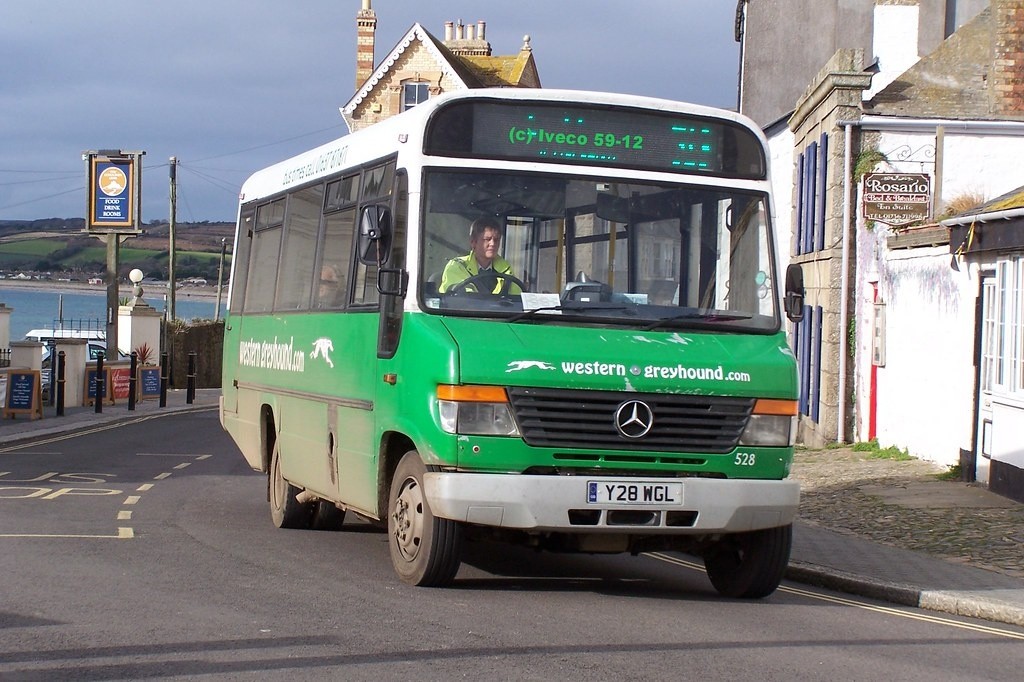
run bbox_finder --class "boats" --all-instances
[20,329,106,354]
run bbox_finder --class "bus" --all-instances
[219,86,805,600]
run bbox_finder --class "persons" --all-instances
[440,218,522,295]
[317,264,348,308]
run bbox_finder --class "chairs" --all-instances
[428,271,443,298]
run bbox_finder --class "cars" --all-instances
[41,341,142,390]
